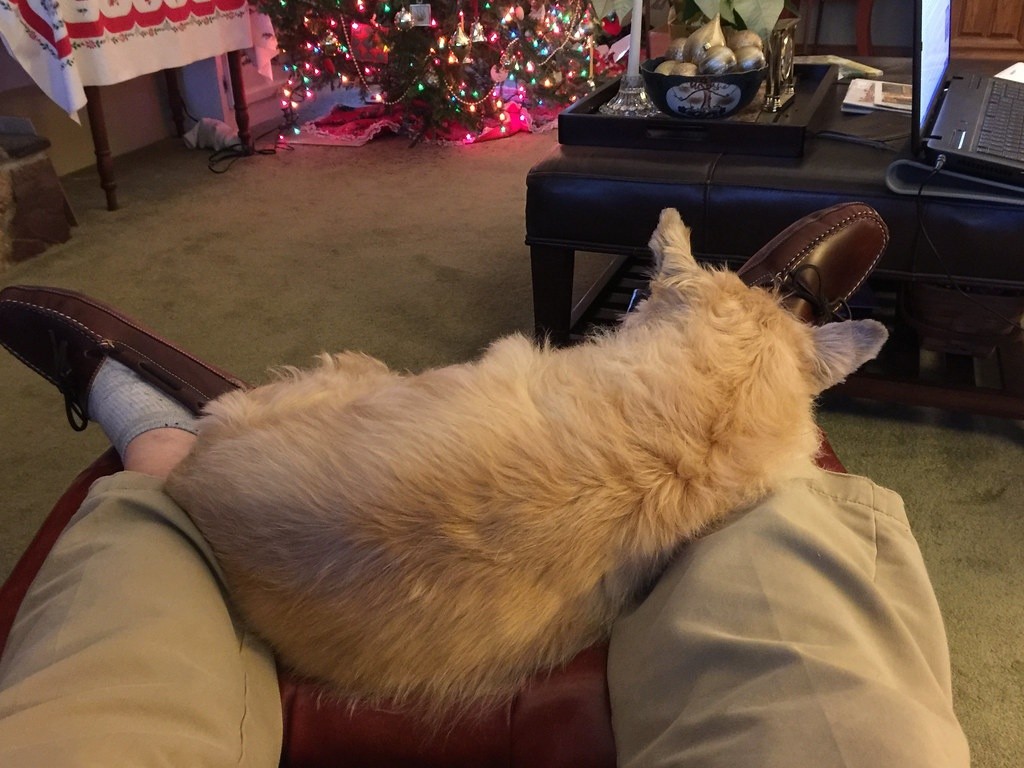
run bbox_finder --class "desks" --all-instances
[0,0,278,212]
[525,55,1024,427]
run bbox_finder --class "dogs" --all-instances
[162,206,890,737]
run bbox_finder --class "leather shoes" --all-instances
[735,199,890,328]
[0,283,256,420]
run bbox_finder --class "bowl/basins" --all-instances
[639,56,769,120]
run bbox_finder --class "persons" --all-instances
[0,201,971,768]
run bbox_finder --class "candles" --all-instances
[626,0,642,75]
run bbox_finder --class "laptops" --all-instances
[911,0,1024,183]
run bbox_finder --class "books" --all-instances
[842,78,913,115]
